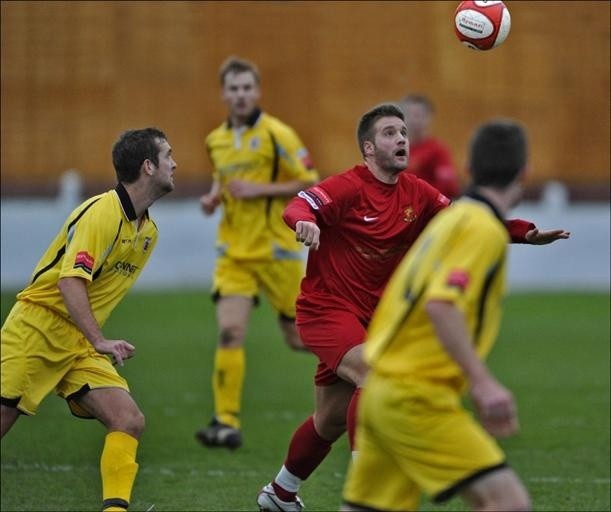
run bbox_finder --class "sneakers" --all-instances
[257,483,306,512]
[195,416,242,449]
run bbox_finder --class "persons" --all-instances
[403,95,460,203]
[195,58,321,447]
[341,118,533,512]
[0,127,178,512]
[256,104,570,512]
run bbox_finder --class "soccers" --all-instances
[452,0,512,51]
